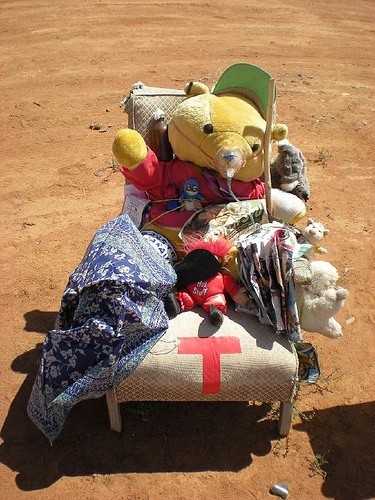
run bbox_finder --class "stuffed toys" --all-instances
[178,178,205,211]
[112,64,306,257]
[294,261,349,338]
[270,143,310,207]
[165,233,256,328]
[298,219,330,256]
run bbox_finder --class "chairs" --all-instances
[104,79,300,438]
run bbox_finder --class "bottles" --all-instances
[151,118,173,161]
